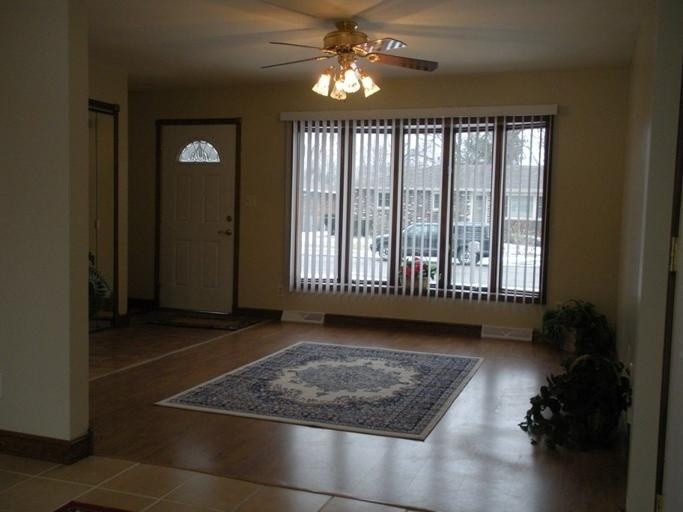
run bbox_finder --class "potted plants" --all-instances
[532,298,617,353]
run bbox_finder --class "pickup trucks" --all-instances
[369,222,490,265]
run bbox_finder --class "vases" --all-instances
[400,274,428,290]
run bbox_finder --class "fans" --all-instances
[261,21,439,72]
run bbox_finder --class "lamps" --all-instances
[311,60,381,101]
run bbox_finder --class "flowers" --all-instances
[397,255,437,281]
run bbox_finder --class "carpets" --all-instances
[143,315,257,331]
[153,339,484,441]
